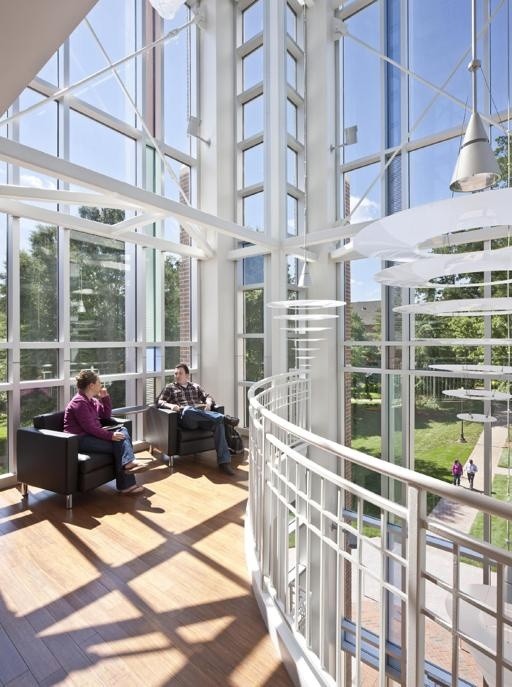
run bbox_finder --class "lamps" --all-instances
[448,1,501,192]
[149,0,187,20]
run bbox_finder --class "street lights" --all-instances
[459,385,467,442]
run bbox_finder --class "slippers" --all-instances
[116,484,146,497]
[120,460,144,472]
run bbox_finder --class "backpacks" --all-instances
[223,425,244,455]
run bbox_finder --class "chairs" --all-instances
[16,408,132,510]
[143,400,224,468]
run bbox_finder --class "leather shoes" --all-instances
[222,415,240,426]
[218,463,236,475]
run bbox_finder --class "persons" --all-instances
[156,362,240,475]
[452,458,462,485]
[465,459,477,489]
[62,369,146,496]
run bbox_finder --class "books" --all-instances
[100,423,125,431]
